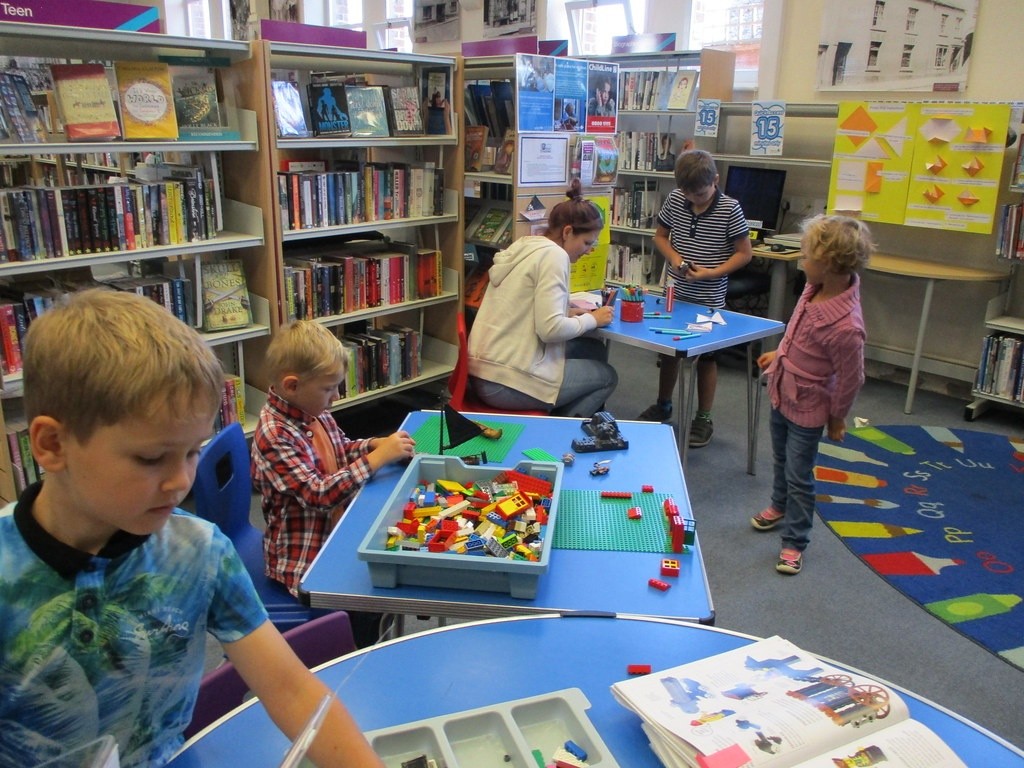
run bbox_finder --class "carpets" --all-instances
[813,425,1024,672]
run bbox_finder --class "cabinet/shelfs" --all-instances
[0,19,1024,502]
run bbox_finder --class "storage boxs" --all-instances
[357,454,564,599]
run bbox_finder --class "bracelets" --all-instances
[678,261,689,273]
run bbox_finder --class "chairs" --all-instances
[192,423,378,658]
[447,312,549,416]
[183,611,358,740]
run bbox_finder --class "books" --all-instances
[604,65,701,294]
[269,65,454,138]
[333,319,427,402]
[0,151,224,264]
[995,135,1024,263]
[283,238,441,322]
[202,367,249,437]
[0,53,242,144]
[277,154,449,231]
[4,409,50,502]
[976,334,1024,402]
[1,256,255,332]
[463,76,517,313]
[610,634,967,768]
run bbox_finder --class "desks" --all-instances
[297,410,715,636]
[566,288,786,477]
[862,253,1012,415]
[167,613,1024,768]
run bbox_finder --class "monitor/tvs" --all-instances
[725,166,787,238]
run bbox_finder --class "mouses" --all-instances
[770,243,786,252]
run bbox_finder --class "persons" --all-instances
[675,76,689,97]
[587,72,618,117]
[1,286,388,768]
[558,103,580,131]
[250,321,417,647]
[495,140,515,173]
[464,178,619,416]
[638,149,753,446]
[656,136,675,172]
[751,212,874,575]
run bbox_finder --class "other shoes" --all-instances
[751,507,785,530]
[776,548,803,574]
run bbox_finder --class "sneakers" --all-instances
[688,417,715,446]
[633,405,673,424]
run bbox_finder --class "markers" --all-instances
[601,279,619,306]
[619,283,648,301]
[643,315,672,318]
[649,327,685,331]
[674,334,701,340]
[596,302,601,308]
[655,331,692,334]
[643,312,660,314]
